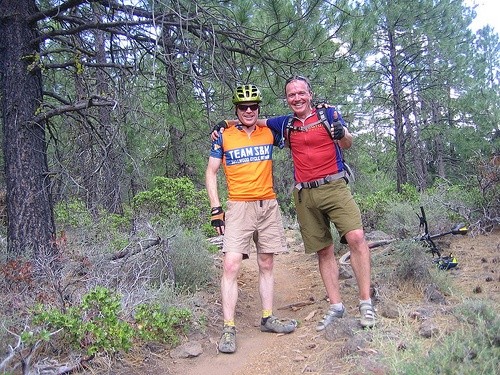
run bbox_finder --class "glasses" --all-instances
[237,104,259,111]
[285,76,311,88]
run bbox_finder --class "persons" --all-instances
[204,83,337,352]
[210,75,376,332]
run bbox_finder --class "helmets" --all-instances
[232,84,263,104]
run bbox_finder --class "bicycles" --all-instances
[338,205,470,271]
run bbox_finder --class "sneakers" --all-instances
[219,326,236,352]
[261,315,294,332]
[359,301,375,326]
[316,304,347,331]
